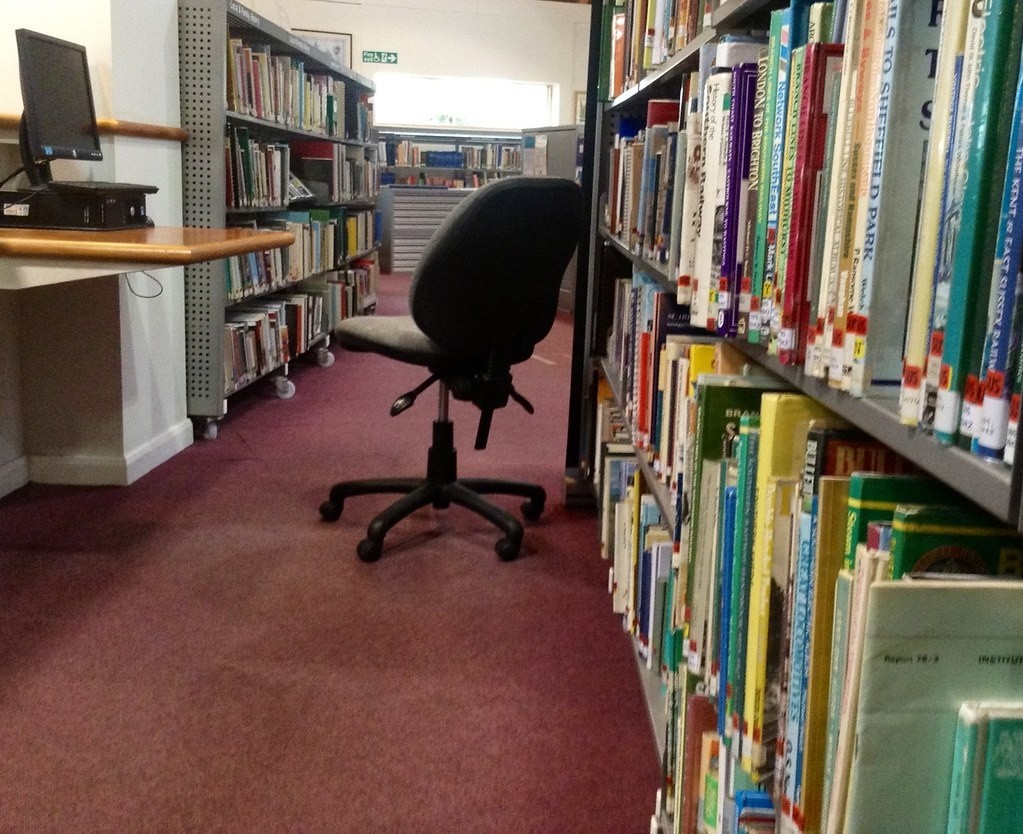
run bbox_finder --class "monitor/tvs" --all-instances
[15,28,104,190]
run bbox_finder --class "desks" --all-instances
[0,223,296,499]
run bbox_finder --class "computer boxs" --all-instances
[0,189,150,232]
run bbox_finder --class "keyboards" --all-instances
[48,180,159,193]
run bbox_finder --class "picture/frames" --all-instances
[291,27,353,68]
[574,90,585,124]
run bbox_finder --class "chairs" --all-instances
[318,173,586,561]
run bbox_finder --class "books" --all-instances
[576,0,1023,834]
[224,22,527,392]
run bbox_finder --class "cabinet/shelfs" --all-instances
[178,0,378,440]
[586,0,1023,834]
[375,124,522,185]
